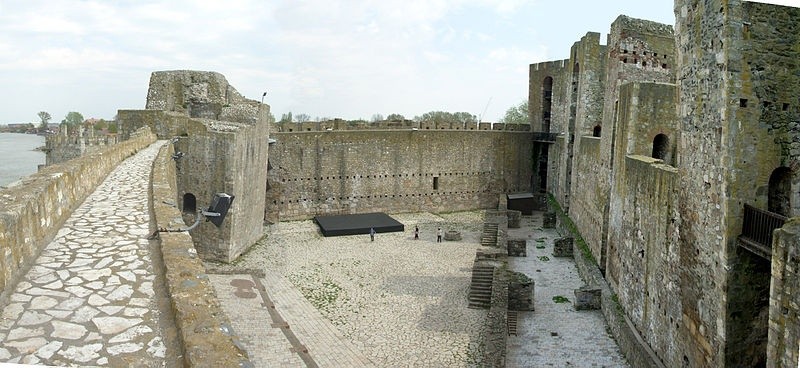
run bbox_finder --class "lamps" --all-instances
[170,137,178,144]
[147,192,236,240]
[170,152,185,161]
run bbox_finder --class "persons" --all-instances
[370,228,376,241]
[437,228,442,243]
[414,225,420,240]
